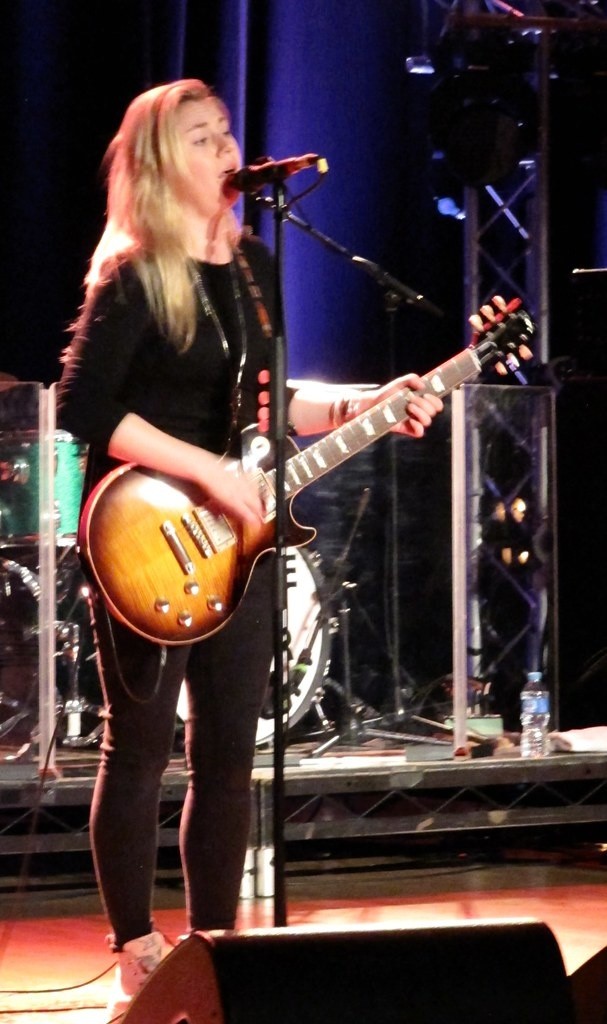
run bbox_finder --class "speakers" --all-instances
[117,921,607,1024]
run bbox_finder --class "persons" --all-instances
[53,81,483,996]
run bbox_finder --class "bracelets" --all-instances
[331,388,359,428]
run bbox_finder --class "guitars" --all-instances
[80,294,536,647]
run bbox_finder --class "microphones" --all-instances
[229,153,325,194]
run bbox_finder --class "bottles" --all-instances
[521,671,551,757]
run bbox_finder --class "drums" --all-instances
[1,427,84,548]
[172,545,334,748]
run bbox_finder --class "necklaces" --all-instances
[185,255,249,450]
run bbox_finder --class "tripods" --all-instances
[286,218,501,764]
[0,552,107,768]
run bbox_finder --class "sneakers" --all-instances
[117,933,176,996]
